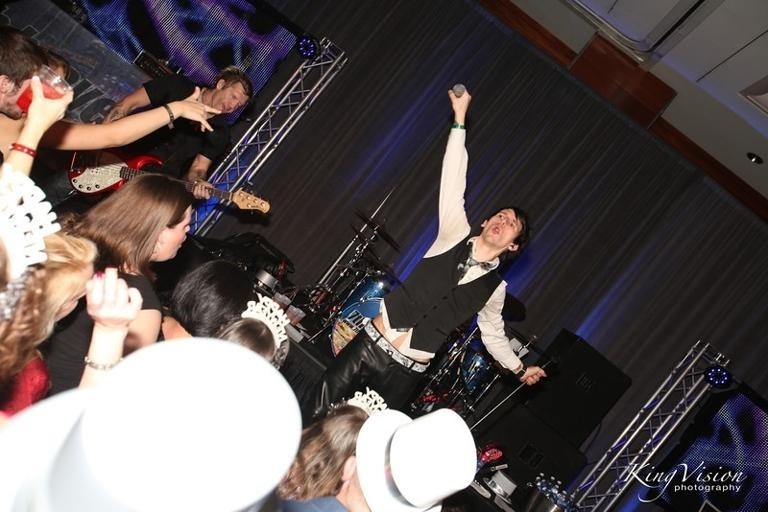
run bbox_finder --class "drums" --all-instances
[332,280,392,356]
[458,352,494,395]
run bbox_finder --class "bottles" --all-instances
[534,472,574,510]
[473,458,486,475]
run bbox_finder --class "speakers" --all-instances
[479,401,588,498]
[525,328,632,444]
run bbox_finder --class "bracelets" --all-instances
[516,366,527,379]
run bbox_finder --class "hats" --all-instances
[355,407,478,512]
[1,337,303,512]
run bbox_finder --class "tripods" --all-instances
[284,227,472,412]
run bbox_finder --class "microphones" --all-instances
[453,83,465,98]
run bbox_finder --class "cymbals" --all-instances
[350,209,399,261]
[510,328,550,360]
[376,263,401,285]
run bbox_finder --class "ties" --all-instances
[458,257,479,281]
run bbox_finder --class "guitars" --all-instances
[68,149,270,214]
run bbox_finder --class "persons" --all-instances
[299,86,548,433]
[0,30,478,512]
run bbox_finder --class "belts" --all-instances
[364,319,431,373]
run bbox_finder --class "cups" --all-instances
[272,292,307,326]
[17,62,75,114]
